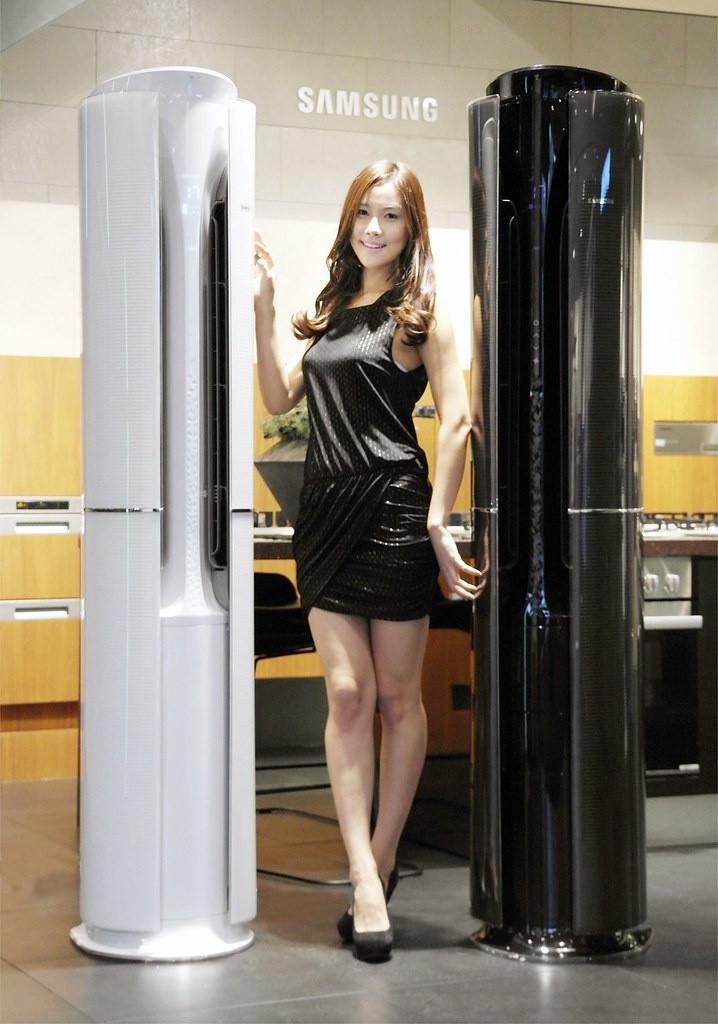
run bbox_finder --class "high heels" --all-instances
[351,875,393,960]
[337,864,400,944]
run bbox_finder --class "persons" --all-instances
[250,158,492,962]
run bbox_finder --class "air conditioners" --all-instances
[71,65,258,963]
[465,66,644,963]
